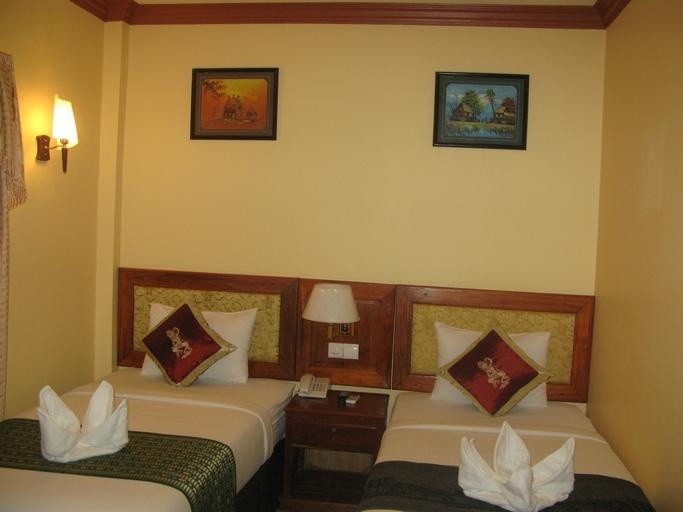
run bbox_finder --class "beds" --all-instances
[362,387,652,512]
[1,366,297,512]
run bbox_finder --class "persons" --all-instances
[223,93,258,123]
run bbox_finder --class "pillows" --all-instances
[143,298,259,385]
[429,317,552,408]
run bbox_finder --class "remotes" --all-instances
[346,394,360,404]
[338,391,350,399]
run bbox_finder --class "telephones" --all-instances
[298,373,330,398]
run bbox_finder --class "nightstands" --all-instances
[281,387,389,511]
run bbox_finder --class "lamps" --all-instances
[301,281,361,340]
[32,95,77,171]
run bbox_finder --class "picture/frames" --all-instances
[191,67,278,141]
[432,69,528,151]
